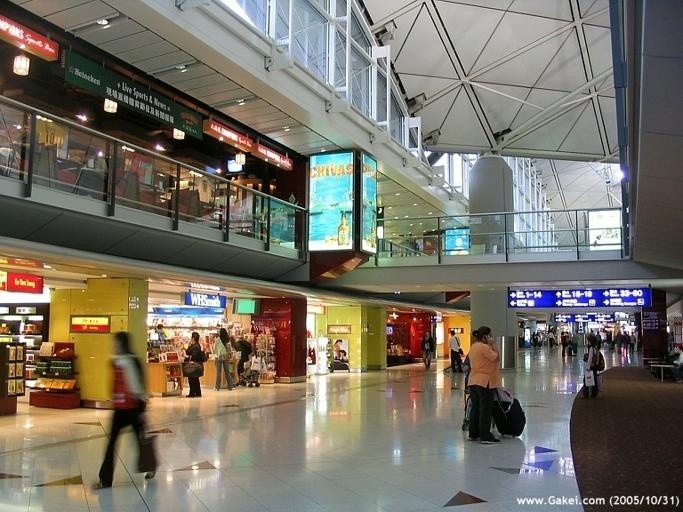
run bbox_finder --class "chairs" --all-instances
[10,143,222,225]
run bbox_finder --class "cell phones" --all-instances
[484,336,488,339]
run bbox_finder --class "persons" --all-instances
[148,326,157,340]
[154,324,165,339]
[397,233,421,256]
[449,331,463,374]
[531,327,635,358]
[229,334,252,387]
[666,332,682,384]
[332,339,342,360]
[200,180,208,202]
[180,332,205,398]
[213,327,236,391]
[420,331,433,369]
[577,333,599,400]
[339,350,347,360]
[466,327,506,446]
[91,332,159,490]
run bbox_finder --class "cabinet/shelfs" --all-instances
[0,303,81,416]
[199,359,240,389]
[144,362,184,397]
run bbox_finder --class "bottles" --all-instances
[370,215,376,248]
[337,209,351,246]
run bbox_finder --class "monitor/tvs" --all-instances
[431,315,442,323]
[232,298,261,315]
[224,158,246,176]
[387,325,394,334]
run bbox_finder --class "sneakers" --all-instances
[480,438,500,444]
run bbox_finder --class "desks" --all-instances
[642,358,676,383]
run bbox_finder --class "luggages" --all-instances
[491,398,525,439]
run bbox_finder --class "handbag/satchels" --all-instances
[584,353,597,363]
[459,349,463,355]
[493,388,513,402]
[182,361,204,377]
[595,351,605,371]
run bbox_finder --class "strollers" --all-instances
[459,372,472,431]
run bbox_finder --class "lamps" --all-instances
[173,127,186,140]
[104,98,118,113]
[235,150,246,165]
[13,51,30,75]
